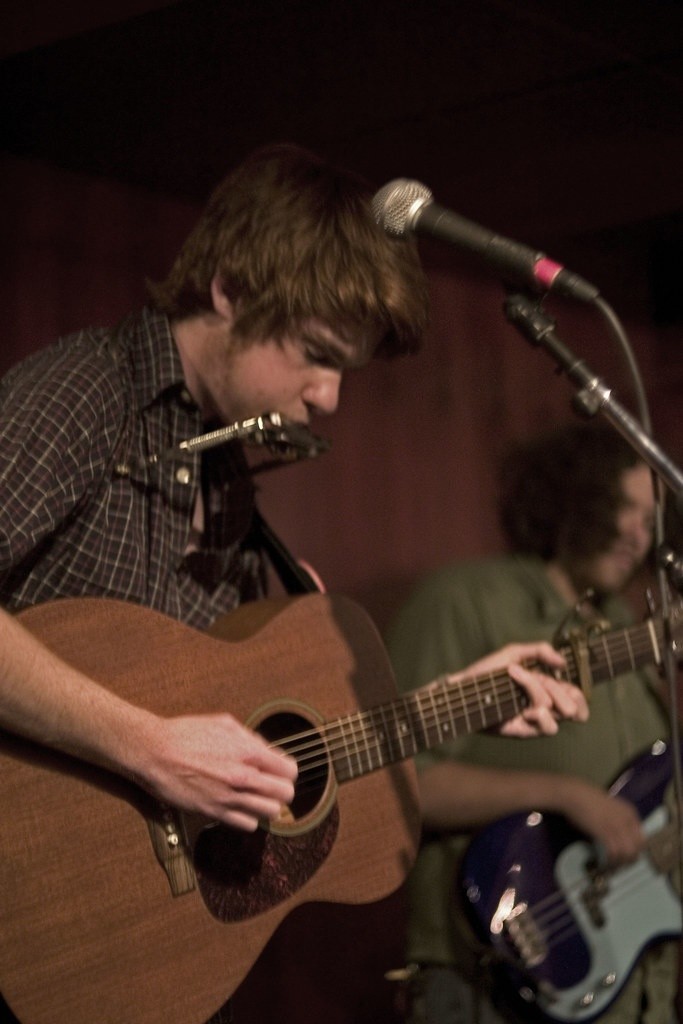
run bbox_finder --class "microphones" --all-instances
[370,179,601,305]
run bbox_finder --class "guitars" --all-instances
[453,736,683,1024]
[1,587,683,1024]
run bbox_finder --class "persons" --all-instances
[0,142,592,830]
[385,424,683,1024]
